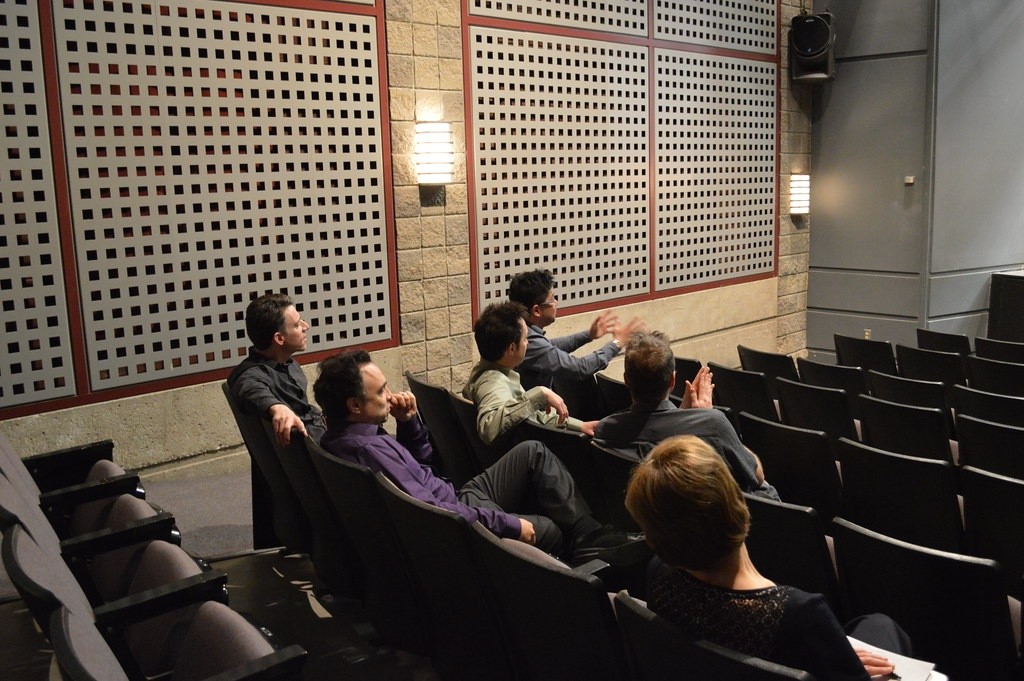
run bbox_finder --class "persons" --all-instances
[229,292,327,447]
[595,330,784,504]
[462,300,601,443]
[624,437,898,680]
[508,270,647,417]
[312,347,657,567]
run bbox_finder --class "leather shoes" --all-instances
[572,526,647,569]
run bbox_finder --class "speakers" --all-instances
[791,13,837,83]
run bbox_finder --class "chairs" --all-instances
[3,249,1024,681]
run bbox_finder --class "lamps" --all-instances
[410,120,457,190]
[787,172,813,218]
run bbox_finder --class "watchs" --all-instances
[610,339,624,350]
